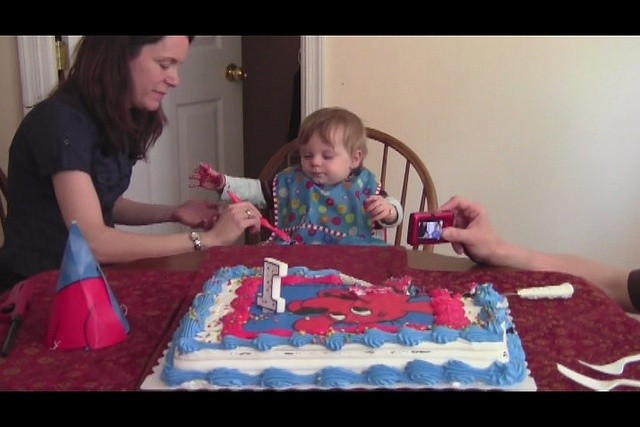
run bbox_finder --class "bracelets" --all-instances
[189,231,202,251]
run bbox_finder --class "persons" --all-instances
[435,195,639,315]
[1,35,261,290]
[189,107,403,247]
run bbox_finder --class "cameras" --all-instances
[406,211,457,246]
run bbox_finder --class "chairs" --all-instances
[0,163,15,248]
[241,128,438,253]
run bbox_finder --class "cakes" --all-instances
[161,264,528,386]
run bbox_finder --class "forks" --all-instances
[576,354,640,377]
[556,362,640,392]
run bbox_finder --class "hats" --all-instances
[45,221,129,353]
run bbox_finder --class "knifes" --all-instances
[500,281,575,300]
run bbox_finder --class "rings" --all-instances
[245,209,251,217]
